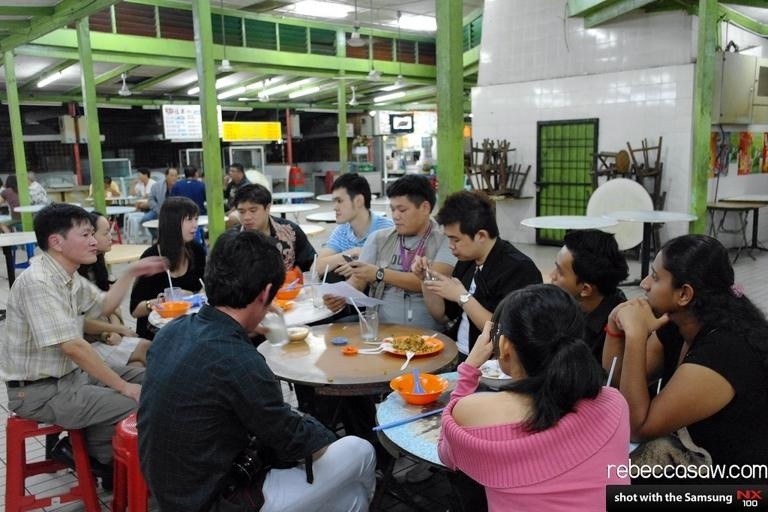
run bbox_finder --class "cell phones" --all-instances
[342,253,357,268]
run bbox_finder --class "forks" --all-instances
[400,350,414,371]
[359,337,395,354]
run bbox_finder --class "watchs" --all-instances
[456,292,472,308]
[375,268,386,283]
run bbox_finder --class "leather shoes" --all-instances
[49,439,98,485]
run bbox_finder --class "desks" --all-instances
[1,180,387,309]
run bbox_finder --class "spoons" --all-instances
[413,368,425,393]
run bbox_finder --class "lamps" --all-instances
[366,0,384,81]
[346,0,369,47]
[215,1,234,73]
[1,53,364,109]
[390,7,410,88]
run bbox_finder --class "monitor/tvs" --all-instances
[391,114,414,133]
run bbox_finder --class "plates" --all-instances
[384,336,445,357]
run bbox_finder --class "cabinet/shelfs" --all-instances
[584,178,659,252]
[711,47,722,125]
[751,58,768,125]
[726,193,768,252]
[520,209,618,244]
[721,52,759,126]
[602,206,701,291]
[710,201,761,267]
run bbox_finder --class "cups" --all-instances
[157,287,182,304]
[311,283,327,309]
[262,306,291,348]
[303,271,318,299]
[359,310,379,341]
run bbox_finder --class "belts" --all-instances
[5,377,56,389]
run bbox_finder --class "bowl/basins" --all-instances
[157,302,190,318]
[276,283,302,300]
[286,324,311,343]
[284,270,298,283]
[480,360,513,390]
[388,373,449,404]
[421,273,450,283]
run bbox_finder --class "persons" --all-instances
[1,164,255,255]
[602,233,768,509]
[323,174,458,330]
[548,226,628,371]
[129,194,206,342]
[435,281,633,511]
[73,212,155,367]
[1,203,169,496]
[314,174,395,279]
[408,187,543,374]
[135,228,376,510]
[228,184,317,272]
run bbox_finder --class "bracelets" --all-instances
[602,323,625,340]
[145,300,153,314]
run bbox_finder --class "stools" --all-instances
[460,128,531,199]
[108,403,158,511]
[5,412,101,511]
[590,130,673,260]
